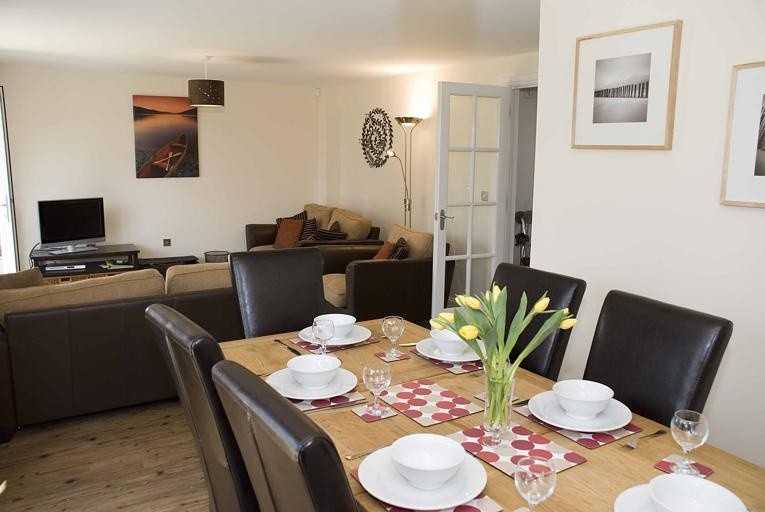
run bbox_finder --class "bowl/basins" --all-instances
[430,328,468,355]
[390,433,466,490]
[314,314,356,340]
[286,354,342,390]
[649,474,726,511]
[552,380,615,420]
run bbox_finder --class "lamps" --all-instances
[386,116,423,228]
[187,79,225,108]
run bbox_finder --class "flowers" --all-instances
[431,288,577,379]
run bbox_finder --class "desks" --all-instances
[31,244,140,277]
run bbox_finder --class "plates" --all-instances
[416,338,487,362]
[357,445,488,510]
[266,368,358,400]
[299,325,372,346]
[614,483,748,511]
[528,390,633,432]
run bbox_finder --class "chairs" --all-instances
[211,359,362,511]
[145,300,261,512]
[230,248,328,338]
[582,288,735,429]
[488,265,586,380]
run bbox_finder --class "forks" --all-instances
[616,429,668,448]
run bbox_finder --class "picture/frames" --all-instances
[720,61,765,207]
[571,20,682,151]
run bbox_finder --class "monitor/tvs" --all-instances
[37,196,106,254]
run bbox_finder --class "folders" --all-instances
[104,257,134,270]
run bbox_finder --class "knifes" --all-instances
[512,399,531,407]
[274,339,301,356]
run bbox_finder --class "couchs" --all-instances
[314,244,456,322]
[5,262,246,432]
[0,325,19,444]
[245,208,384,251]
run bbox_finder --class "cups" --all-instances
[515,456,557,511]
[381,316,404,357]
[312,320,335,356]
[362,362,391,417]
[670,409,708,474]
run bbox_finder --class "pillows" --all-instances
[273,220,304,248]
[276,210,306,230]
[389,238,409,258]
[0,268,46,289]
[310,229,347,241]
[300,218,316,238]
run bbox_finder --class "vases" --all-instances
[483,379,516,432]
